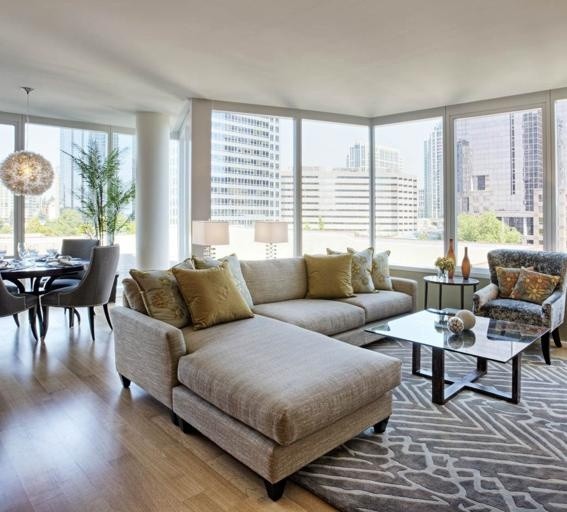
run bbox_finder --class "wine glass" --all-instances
[16,241,39,259]
[0,244,8,260]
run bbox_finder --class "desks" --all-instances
[423,275,480,316]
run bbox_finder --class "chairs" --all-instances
[474,249,567,372]
[0,237,123,347]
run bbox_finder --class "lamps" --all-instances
[190,220,231,259]
[1,85,55,199]
[252,221,289,255]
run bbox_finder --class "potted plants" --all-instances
[53,130,136,303]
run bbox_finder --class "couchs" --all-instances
[108,256,420,500]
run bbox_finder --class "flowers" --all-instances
[435,256,455,272]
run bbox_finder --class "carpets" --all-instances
[257,317,566,511]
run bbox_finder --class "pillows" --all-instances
[303,253,356,299]
[325,247,381,294]
[509,264,560,306]
[494,264,534,300]
[121,249,257,331]
[346,246,398,292]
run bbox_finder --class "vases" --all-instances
[439,269,446,278]
